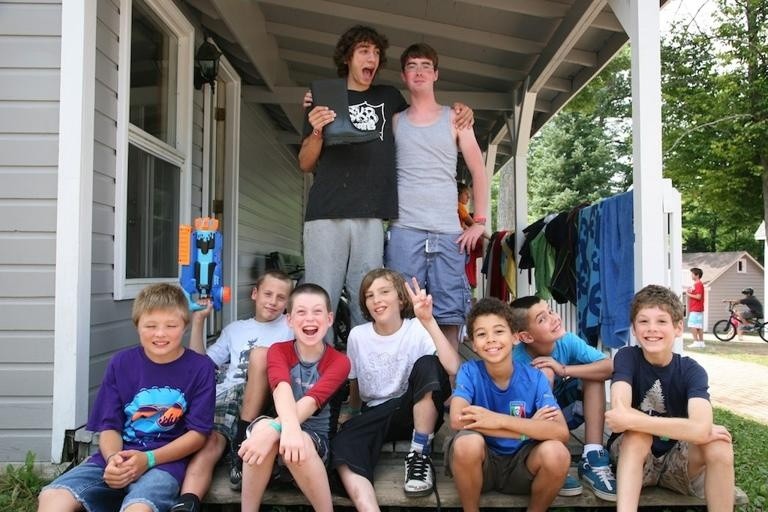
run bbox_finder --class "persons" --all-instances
[331,269,459,511]
[382,44,488,354]
[175,272,293,512]
[441,298,570,511]
[36,285,215,512]
[453,182,480,288]
[605,287,734,512]
[510,296,620,502]
[297,25,408,355]
[685,270,708,349]
[237,284,350,512]
[721,288,763,334]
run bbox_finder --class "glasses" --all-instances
[401,62,437,73]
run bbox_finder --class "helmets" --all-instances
[742,287,755,294]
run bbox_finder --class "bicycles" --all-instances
[713,301,768,342]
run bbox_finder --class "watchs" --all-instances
[472,214,487,224]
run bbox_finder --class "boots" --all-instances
[309,78,380,148]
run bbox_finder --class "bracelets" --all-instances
[519,433,528,442]
[660,435,669,441]
[266,420,281,434]
[145,450,157,468]
[561,364,571,383]
[346,406,362,415]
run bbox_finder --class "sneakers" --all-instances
[228,456,246,491]
[578,447,620,502]
[403,449,437,497]
[741,327,754,334]
[686,342,707,349]
[754,323,763,331]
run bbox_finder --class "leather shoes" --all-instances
[558,475,584,498]
